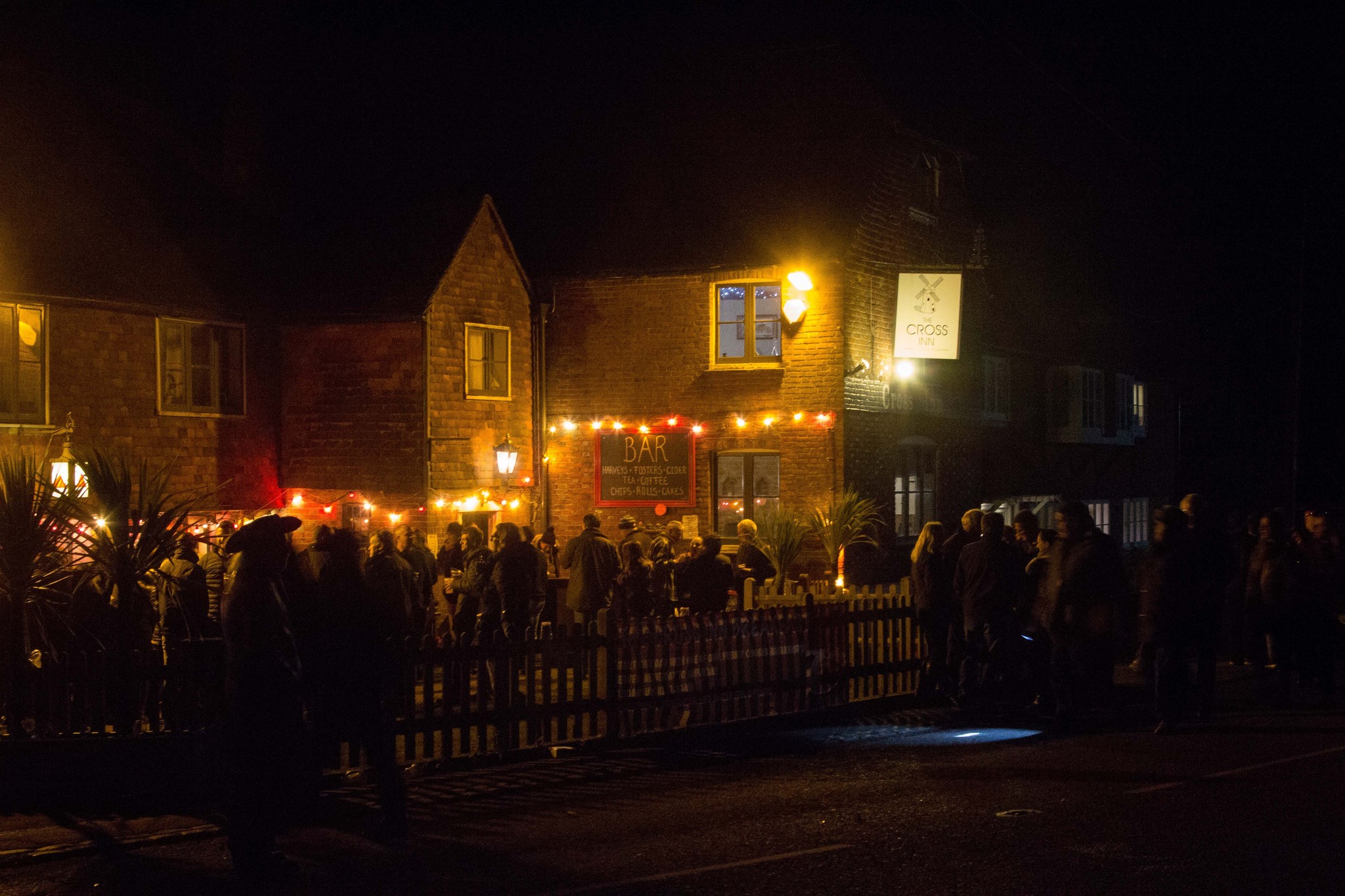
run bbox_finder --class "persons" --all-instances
[226,515,309,884]
[844,482,1345,743]
[311,531,409,840]
[18,501,780,733]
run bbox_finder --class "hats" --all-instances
[541,526,556,545]
[617,515,636,529]
[225,514,301,555]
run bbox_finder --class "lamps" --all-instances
[492,433,521,488]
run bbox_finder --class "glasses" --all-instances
[490,534,500,540]
